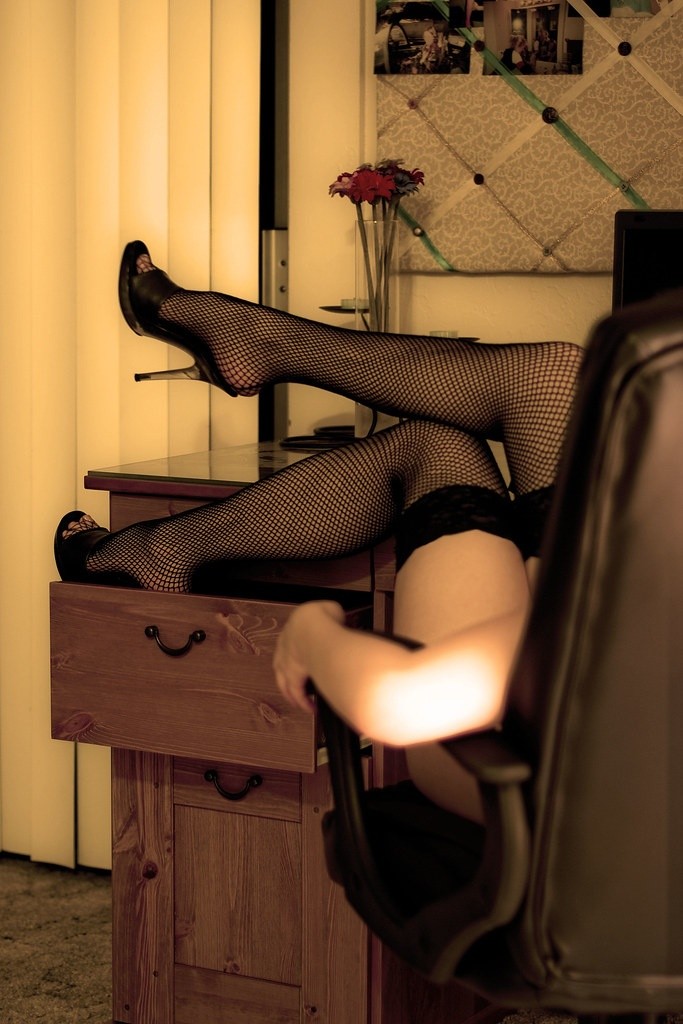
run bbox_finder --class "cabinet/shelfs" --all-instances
[50,438,479,1024]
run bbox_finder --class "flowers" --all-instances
[328,158,426,332]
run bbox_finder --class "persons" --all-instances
[401,27,440,74]
[53,241,585,828]
[488,36,537,75]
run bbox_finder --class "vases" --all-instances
[353,220,398,438]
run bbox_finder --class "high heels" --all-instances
[52,510,143,590]
[119,240,248,397]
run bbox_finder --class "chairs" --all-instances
[307,290,683,1024]
